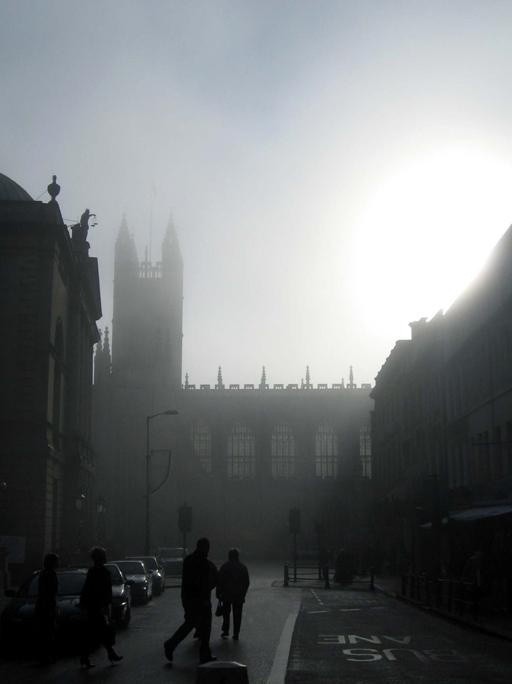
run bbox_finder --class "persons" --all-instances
[216,549,249,640]
[163,538,217,663]
[39,554,58,665]
[80,549,123,668]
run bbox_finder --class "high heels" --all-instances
[107,648,123,665]
[79,656,96,669]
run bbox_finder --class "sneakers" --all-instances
[221,631,240,640]
[199,656,217,663]
[163,641,174,662]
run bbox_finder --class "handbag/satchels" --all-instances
[215,600,224,617]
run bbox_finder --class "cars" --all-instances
[0,548,188,650]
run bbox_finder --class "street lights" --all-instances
[145,410,178,554]
